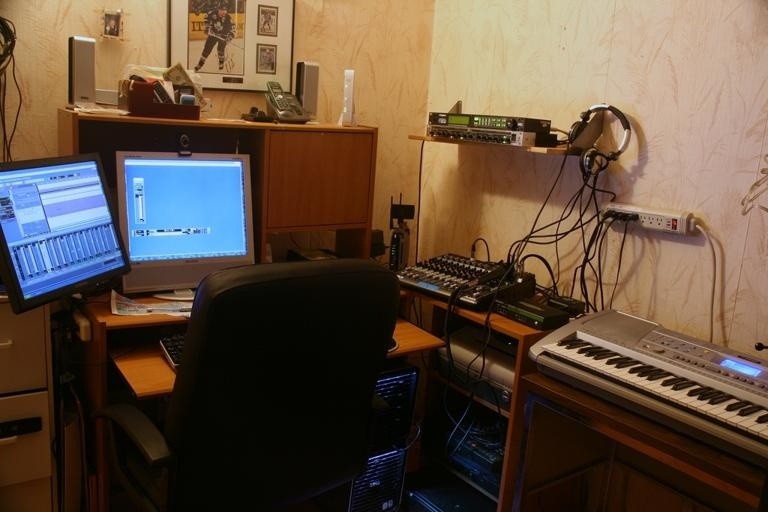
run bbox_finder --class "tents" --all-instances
[568,103,631,175]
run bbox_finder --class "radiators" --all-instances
[153,80,174,104]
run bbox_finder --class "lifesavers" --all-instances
[347,363,419,512]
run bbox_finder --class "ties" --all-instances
[0,153,131,314]
[116,151,256,301]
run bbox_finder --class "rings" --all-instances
[146,307,192,312]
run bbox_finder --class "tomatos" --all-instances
[528,310,768,470]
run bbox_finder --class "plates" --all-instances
[396,278,548,512]
[0,296,57,512]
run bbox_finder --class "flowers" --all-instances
[455,261,513,292]
[755,343,768,351]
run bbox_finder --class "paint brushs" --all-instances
[601,203,692,235]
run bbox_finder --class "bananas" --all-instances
[160,331,188,370]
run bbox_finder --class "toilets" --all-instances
[265,82,311,124]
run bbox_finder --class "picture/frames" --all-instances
[58,106,445,512]
[518,374,768,512]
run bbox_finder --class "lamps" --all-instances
[97,258,401,512]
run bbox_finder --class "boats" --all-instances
[295,61,320,124]
[68,36,95,110]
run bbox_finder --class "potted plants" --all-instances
[97,8,126,43]
[167,0,296,93]
